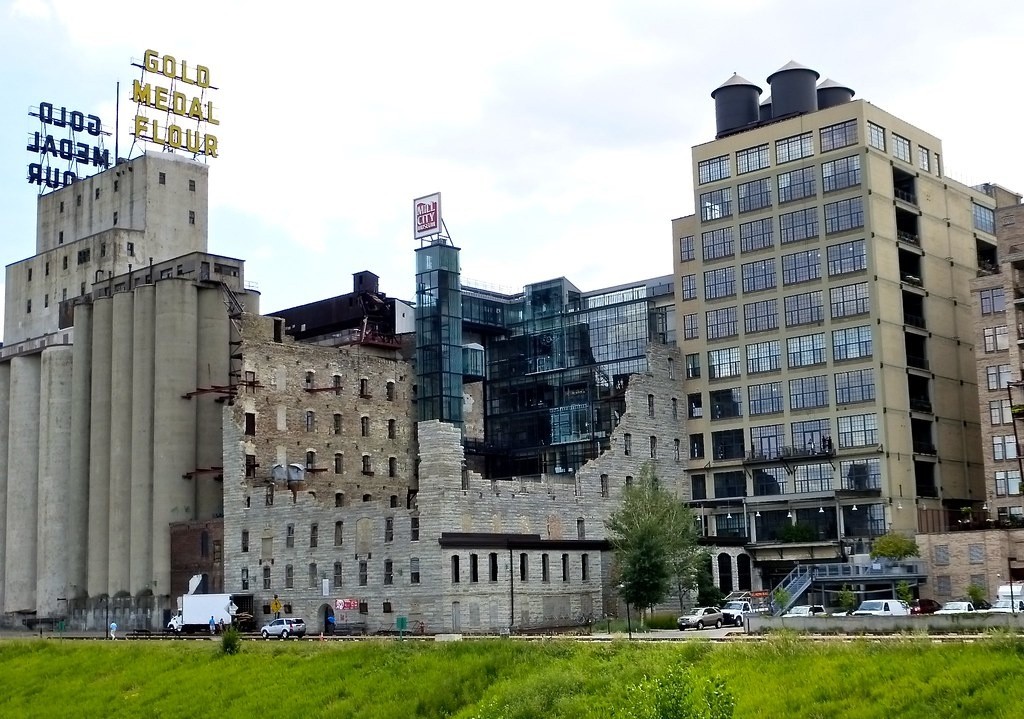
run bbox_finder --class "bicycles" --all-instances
[577,612,597,625]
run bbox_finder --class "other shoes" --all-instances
[114,637,115,640]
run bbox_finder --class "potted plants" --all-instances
[869,532,921,574]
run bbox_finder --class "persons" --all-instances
[208,615,216,636]
[328,613,337,636]
[218,618,225,636]
[109,621,117,641]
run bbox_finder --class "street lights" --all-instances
[99,594,109,638]
[619,581,634,640]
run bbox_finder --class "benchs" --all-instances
[133,629,151,640]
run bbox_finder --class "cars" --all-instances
[987,600,1024,612]
[934,602,978,614]
[910,599,943,614]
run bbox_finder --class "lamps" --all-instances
[897,502,903,510]
[818,507,824,513]
[696,515,701,521]
[851,504,858,510]
[756,511,761,516]
[727,513,732,518]
[983,500,989,510]
[922,503,927,511]
[786,512,793,517]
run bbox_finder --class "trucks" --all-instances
[167,593,253,634]
[997,584,1024,600]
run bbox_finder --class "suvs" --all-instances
[677,607,723,630]
[261,618,306,638]
[721,601,752,626]
[852,600,911,615]
[782,605,827,617]
